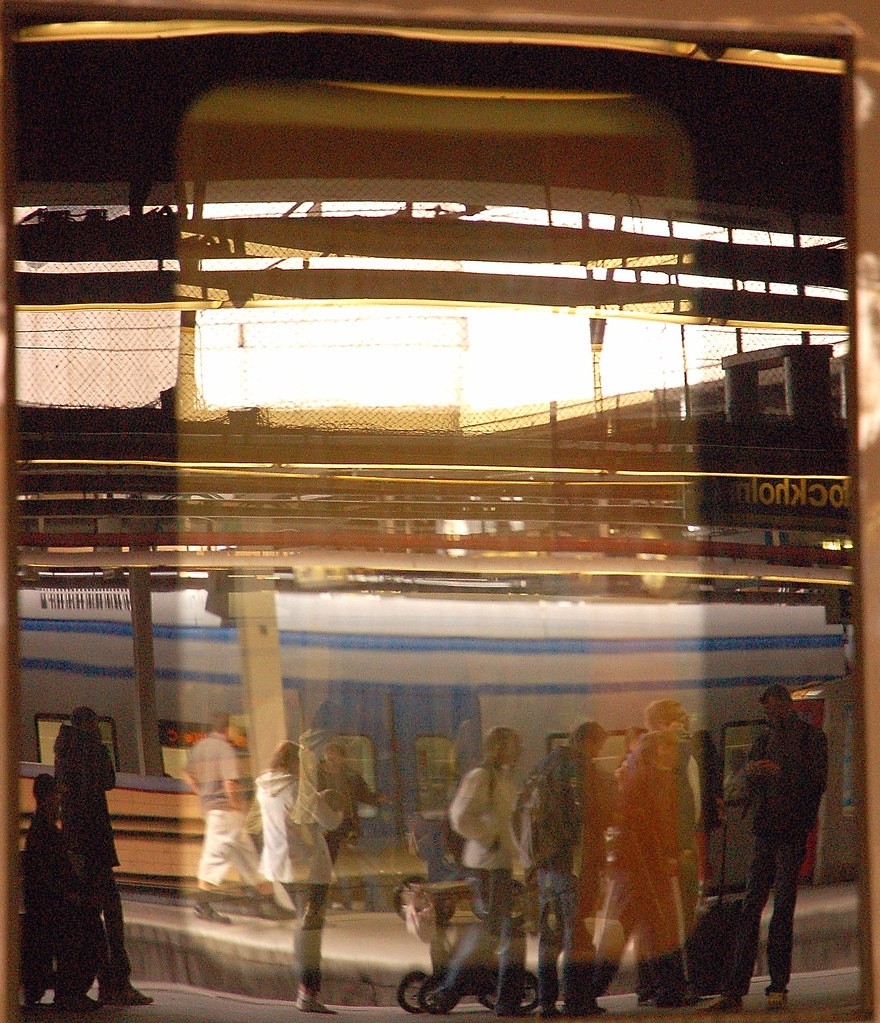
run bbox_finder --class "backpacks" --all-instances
[510,754,578,866]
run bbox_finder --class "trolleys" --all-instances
[396,880,540,1016]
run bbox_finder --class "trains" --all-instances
[9,564,860,844]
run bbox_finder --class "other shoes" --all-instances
[297,995,336,1014]
[767,990,787,1008]
[51,989,98,1011]
[494,1005,536,1016]
[194,904,231,923]
[702,998,741,1012]
[98,985,152,1005]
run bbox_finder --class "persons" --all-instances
[179,705,391,1014]
[435,686,828,1018]
[23,707,154,1014]
